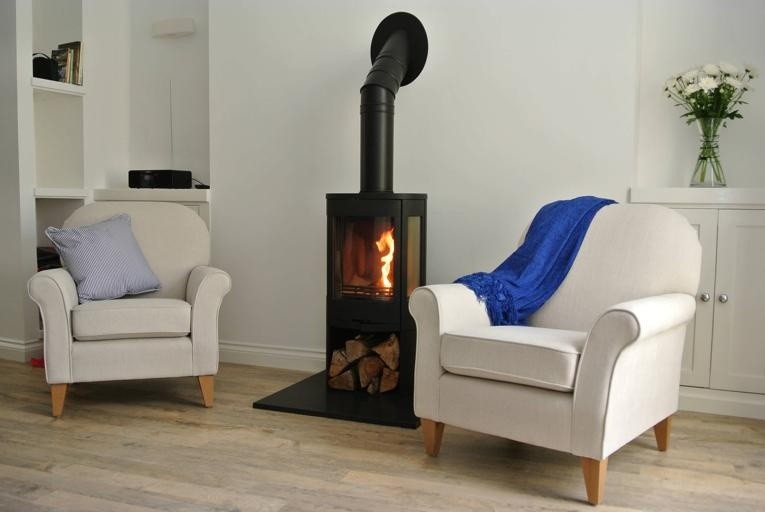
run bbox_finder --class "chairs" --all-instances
[27,201,232,417]
[408,203,703,506]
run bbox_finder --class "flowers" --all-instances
[663,61,757,183]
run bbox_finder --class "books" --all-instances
[50,41,81,84]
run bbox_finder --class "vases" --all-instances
[690,118,726,187]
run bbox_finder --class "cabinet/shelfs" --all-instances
[670,202,765,419]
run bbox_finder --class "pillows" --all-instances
[44,213,161,303]
